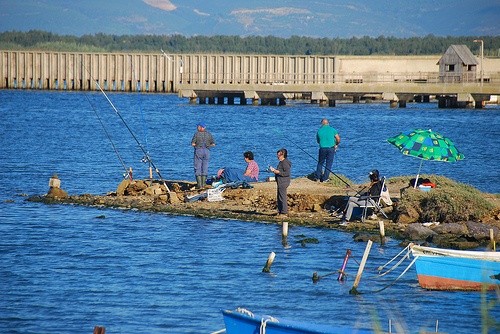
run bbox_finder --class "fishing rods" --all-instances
[71,63,170,194]
[286,137,359,193]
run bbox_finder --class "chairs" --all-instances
[357,176,389,224]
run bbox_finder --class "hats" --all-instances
[196,121,205,128]
[368,169,379,175]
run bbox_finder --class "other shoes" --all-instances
[316,178,330,183]
[274,213,287,218]
[339,221,348,226]
[336,212,344,218]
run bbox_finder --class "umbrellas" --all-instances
[386,129,465,190]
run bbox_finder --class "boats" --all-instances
[219,308,383,334]
[409,243,500,291]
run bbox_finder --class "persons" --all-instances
[243,151,259,182]
[336,169,381,226]
[314,119,340,182]
[192,123,215,188]
[273,149,292,219]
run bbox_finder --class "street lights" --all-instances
[473,39,484,86]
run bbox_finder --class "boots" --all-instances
[194,176,207,188]
